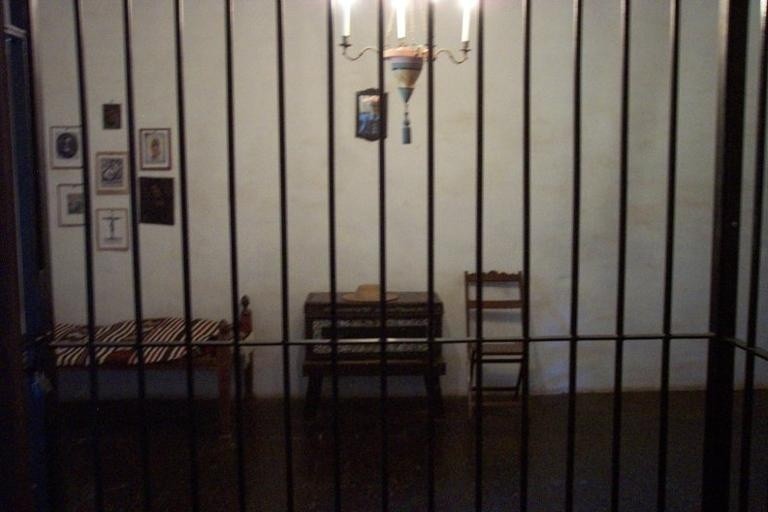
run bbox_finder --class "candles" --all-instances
[331,1,481,43]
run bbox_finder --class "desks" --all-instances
[300,290,447,424]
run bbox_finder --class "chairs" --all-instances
[465,271,534,425]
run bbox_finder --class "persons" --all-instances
[359,94,382,136]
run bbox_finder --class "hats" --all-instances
[342,283,398,302]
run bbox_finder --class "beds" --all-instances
[21,297,256,451]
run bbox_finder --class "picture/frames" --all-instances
[46,101,176,251]
[355,87,388,142]
